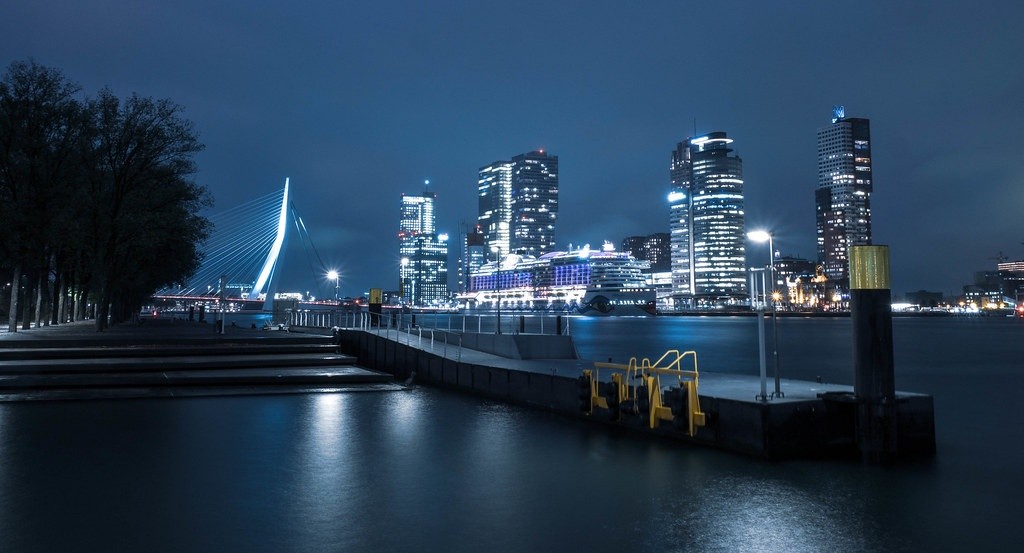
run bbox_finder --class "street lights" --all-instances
[746,226,782,397]
[326,269,339,305]
[400,257,409,295]
[490,245,502,335]
